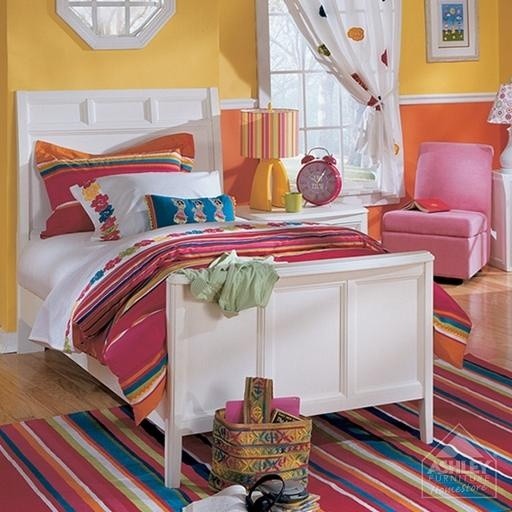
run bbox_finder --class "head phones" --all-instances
[245,473,285,512]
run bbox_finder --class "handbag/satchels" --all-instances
[207,377,312,494]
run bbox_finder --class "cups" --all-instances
[285,192,302,214]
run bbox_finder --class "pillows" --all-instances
[32,131,236,243]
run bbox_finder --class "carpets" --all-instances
[2,352,511,512]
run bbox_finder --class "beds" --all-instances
[13,87,433,489]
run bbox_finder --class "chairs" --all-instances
[381,140,494,284]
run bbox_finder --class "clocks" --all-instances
[296,146,343,208]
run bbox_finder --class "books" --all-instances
[400,198,451,213]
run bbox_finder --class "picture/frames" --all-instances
[423,1,480,64]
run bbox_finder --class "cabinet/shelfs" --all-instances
[489,169,511,272]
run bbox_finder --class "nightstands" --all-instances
[233,203,369,238]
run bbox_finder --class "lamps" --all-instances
[237,103,302,211]
[486,82,512,169]
